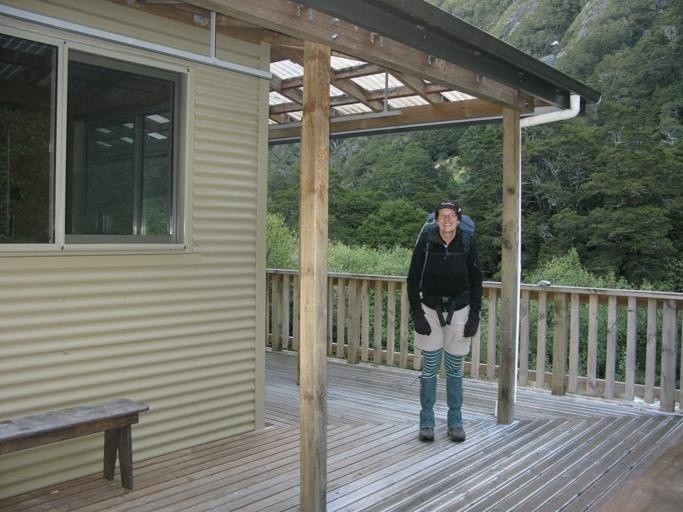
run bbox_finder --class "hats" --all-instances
[435,198,462,220]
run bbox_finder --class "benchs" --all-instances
[1,397,151,491]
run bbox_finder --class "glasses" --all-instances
[438,214,456,218]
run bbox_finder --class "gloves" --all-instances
[464,312,478,338]
[410,311,431,335]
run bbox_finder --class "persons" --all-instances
[407,199,482,441]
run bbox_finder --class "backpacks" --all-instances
[426,212,475,235]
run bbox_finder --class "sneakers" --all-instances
[419,428,434,441]
[449,428,465,440]
